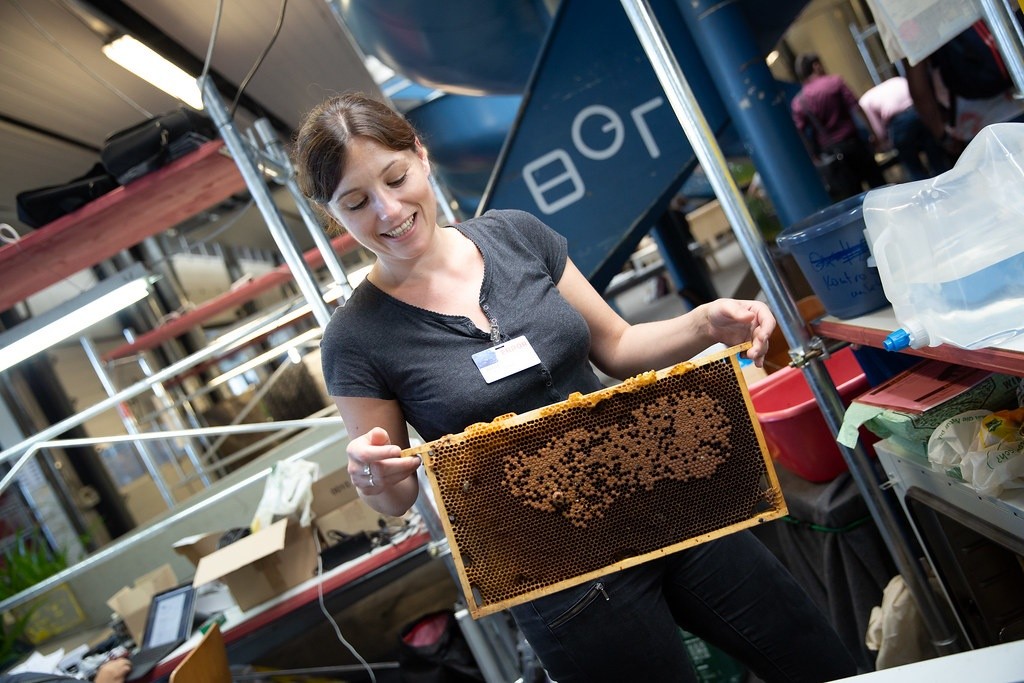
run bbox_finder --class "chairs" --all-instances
[167,622,231,683]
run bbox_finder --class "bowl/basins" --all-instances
[749,345,881,481]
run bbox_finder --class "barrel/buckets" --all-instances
[774,182,896,320]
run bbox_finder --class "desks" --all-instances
[607,242,721,305]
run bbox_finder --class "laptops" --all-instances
[122,580,199,683]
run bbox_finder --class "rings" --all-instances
[369,475,375,487]
[363,464,370,475]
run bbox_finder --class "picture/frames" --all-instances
[9,582,87,647]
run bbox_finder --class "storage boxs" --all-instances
[105,563,179,647]
[751,347,878,482]
[305,461,406,545]
[192,519,318,612]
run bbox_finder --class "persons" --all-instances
[858,77,945,181]
[791,54,886,198]
[294,93,860,683]
[1,657,133,683]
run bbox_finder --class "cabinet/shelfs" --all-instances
[0,75,522,683]
[615,0,1024,656]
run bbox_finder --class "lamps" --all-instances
[100,29,213,110]
[0,261,166,373]
